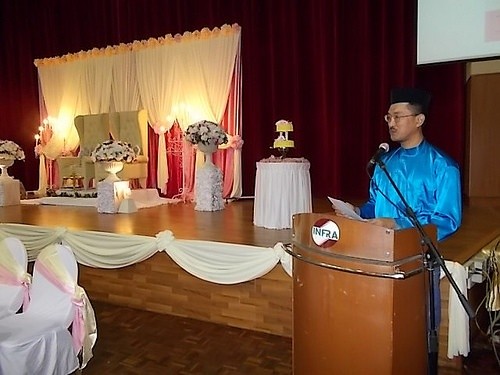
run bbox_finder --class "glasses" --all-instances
[384,113,418,123]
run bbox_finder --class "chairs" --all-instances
[0,237,32,320]
[56,110,148,190]
[0,244,97,375]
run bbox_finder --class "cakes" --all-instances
[275,120,293,131]
[273,136,294,147]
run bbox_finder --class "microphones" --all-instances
[367,143,390,170]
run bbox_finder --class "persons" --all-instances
[332,85,463,375]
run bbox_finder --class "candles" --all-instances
[34,116,56,147]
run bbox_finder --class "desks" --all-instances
[253,161,312,230]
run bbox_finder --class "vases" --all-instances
[100,161,124,174]
[0,159,15,168]
[197,142,218,153]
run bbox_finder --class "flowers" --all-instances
[89,140,137,165]
[0,140,25,161]
[184,120,228,146]
